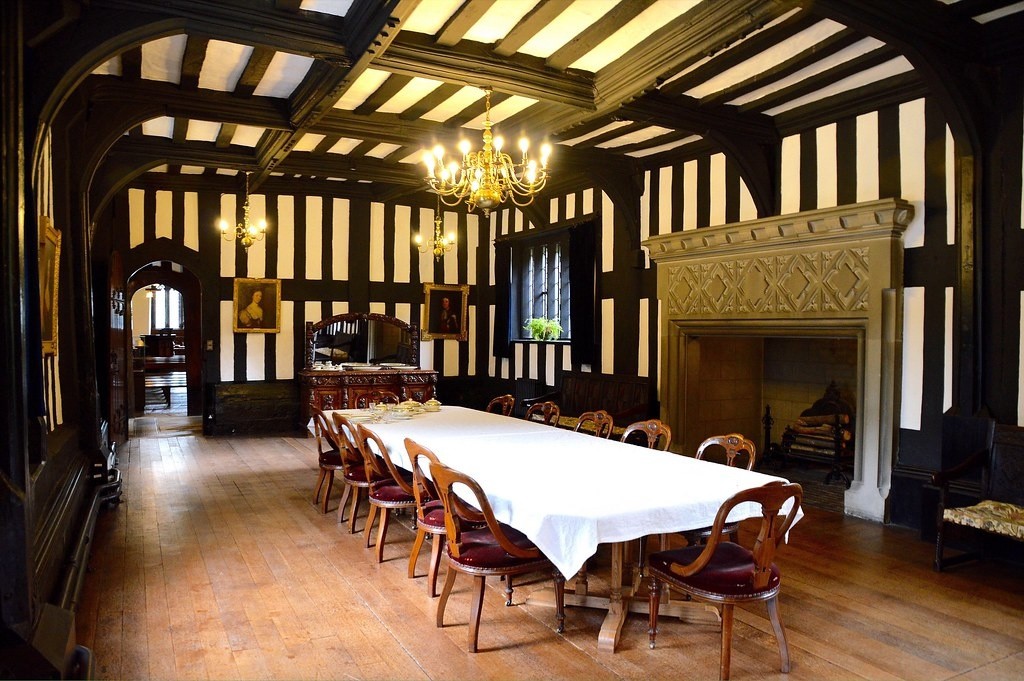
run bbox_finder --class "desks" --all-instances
[309,404,807,653]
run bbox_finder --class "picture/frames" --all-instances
[40,215,62,358]
[422,282,469,342]
[232,278,282,333]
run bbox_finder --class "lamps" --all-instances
[218,171,268,253]
[420,84,553,219]
[145,285,161,293]
[415,194,456,262]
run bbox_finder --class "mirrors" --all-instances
[305,312,420,370]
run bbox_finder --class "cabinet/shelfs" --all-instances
[298,369,439,439]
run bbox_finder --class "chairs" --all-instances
[573,409,613,440]
[527,401,560,426]
[354,388,398,409]
[429,463,566,653]
[621,420,672,453]
[357,423,432,563]
[404,439,483,596]
[680,432,755,550]
[647,481,804,680]
[330,413,393,535]
[931,420,1024,569]
[484,395,514,416]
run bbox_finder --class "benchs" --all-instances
[520,369,655,445]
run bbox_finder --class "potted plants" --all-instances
[525,316,564,341]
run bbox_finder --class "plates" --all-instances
[341,361,373,366]
[313,368,339,371]
[352,367,382,371]
[391,366,418,370]
[337,409,442,424]
[380,362,406,366]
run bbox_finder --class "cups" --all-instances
[314,361,335,369]
[368,398,442,421]
